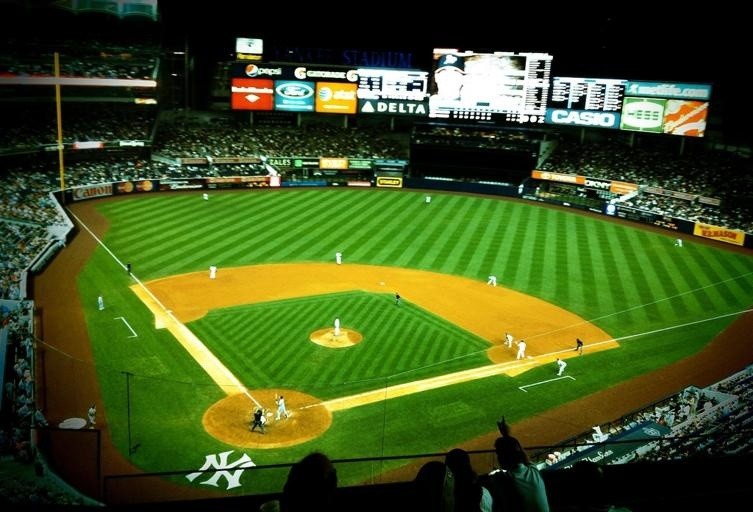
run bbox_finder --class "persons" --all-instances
[395,292,401,305]
[504,333,526,359]
[1,236,99,510]
[677,239,682,247]
[334,317,340,336]
[336,251,342,265]
[126,262,131,276]
[1,38,753,234]
[489,277,496,286]
[251,408,266,432]
[270,371,753,511]
[557,359,567,376]
[210,265,217,280]
[575,338,583,354]
[98,295,105,310]
